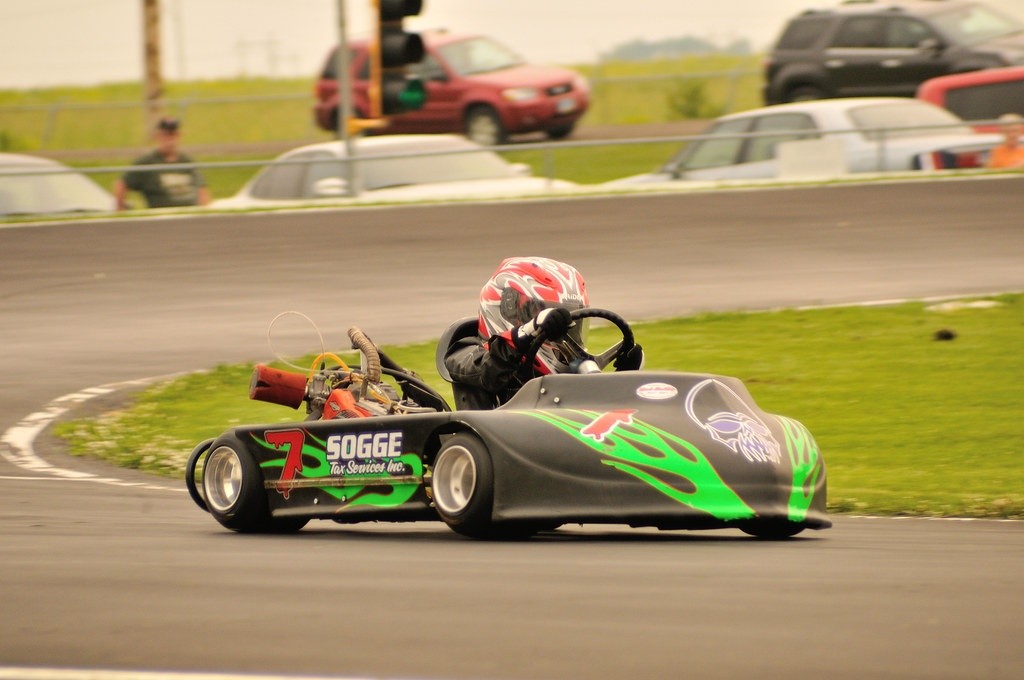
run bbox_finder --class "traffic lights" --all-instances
[371,0,428,121]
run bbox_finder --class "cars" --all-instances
[205,129,579,194]
[607,99,1009,186]
[3,153,129,218]
[314,28,591,142]
[918,67,1024,133]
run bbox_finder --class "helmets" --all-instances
[477,256,590,376]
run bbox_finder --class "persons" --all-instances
[445,254,645,411]
[113,113,212,211]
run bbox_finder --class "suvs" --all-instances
[762,1,1013,105]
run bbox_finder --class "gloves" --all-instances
[523,308,572,344]
[613,343,644,373]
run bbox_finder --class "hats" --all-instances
[157,118,178,130]
